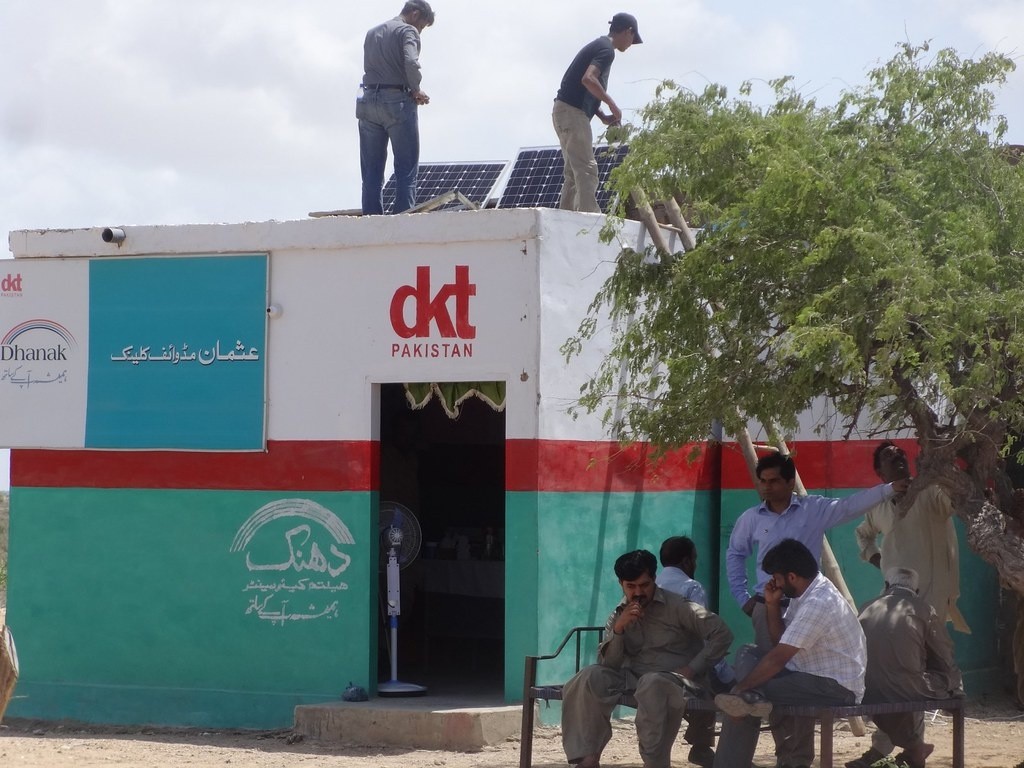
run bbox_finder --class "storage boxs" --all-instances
[426,541,456,560]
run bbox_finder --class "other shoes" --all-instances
[714,691,773,717]
[688,744,715,767]
[844,747,888,768]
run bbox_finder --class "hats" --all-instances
[608,13,643,44]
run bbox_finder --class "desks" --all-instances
[415,559,504,640]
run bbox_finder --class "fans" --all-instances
[379,500,428,697]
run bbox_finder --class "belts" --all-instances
[360,84,409,91]
[754,595,791,609]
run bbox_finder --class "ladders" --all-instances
[627,180,866,742]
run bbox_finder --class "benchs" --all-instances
[520,627,966,768]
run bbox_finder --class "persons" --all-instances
[714,537,868,768]
[726,451,912,768]
[559,549,734,768]
[843,438,972,768]
[551,13,642,216]
[356,0,434,216]
[650,534,717,768]
[852,565,965,768]
